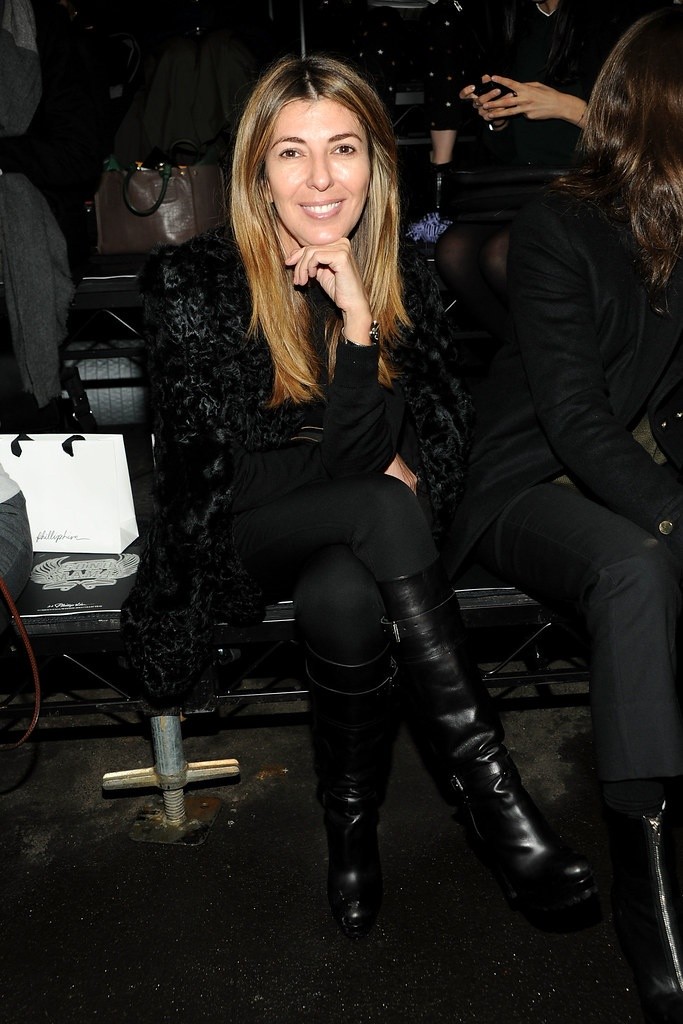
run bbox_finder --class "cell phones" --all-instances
[474,83,507,102]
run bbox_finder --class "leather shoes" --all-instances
[602,784,683,1024]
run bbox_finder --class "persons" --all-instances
[443,6,683,1022]
[116,55,598,936]
[0,0,603,330]
[0,170,76,633]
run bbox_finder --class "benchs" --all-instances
[0,275,595,748]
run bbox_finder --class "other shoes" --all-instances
[422,155,456,218]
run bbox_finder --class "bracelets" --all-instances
[340,321,382,348]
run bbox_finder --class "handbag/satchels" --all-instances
[10,432,140,554]
[96,161,229,255]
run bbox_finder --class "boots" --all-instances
[305,643,401,941]
[381,593,604,920]
